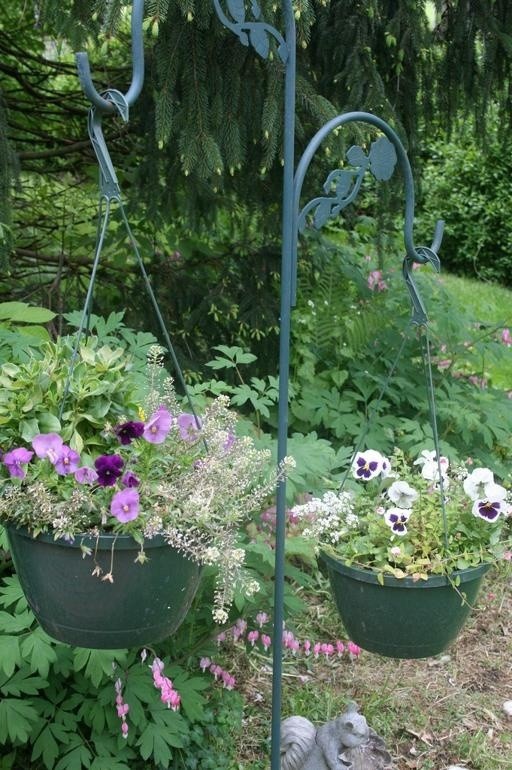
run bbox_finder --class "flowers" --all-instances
[294,439,512,589]
[0,311,283,628]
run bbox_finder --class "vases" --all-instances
[304,545,490,666]
[1,508,228,654]
